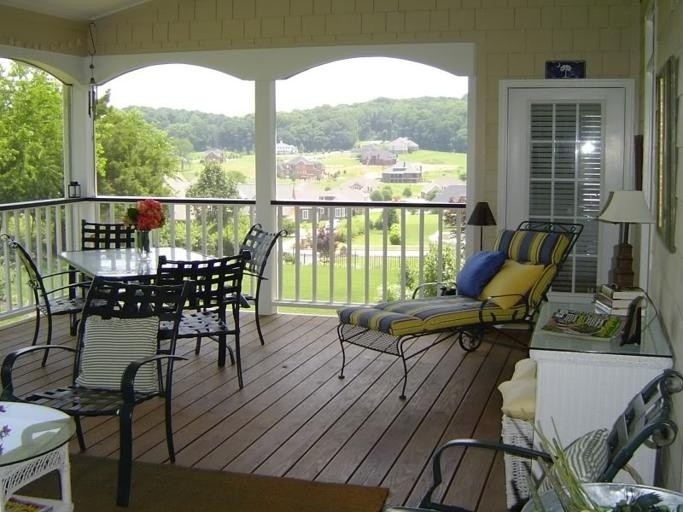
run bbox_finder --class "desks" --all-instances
[0,402,76,512]
[528,301,674,487]
[58,247,236,368]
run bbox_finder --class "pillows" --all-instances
[457,251,504,298]
[478,259,545,310]
[75,315,159,393]
[536,428,610,494]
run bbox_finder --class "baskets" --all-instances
[502,415,533,509]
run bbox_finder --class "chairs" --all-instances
[2,274,190,507]
[383,368,683,512]
[337,221,584,399]
[155,252,251,389]
[68,219,135,336]
[1,234,87,367]
[195,223,287,358]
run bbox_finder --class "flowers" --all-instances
[121,199,165,256]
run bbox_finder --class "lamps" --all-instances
[466,202,496,251]
[596,190,657,289]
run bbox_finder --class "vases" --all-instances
[135,229,150,260]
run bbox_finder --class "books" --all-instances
[601,283,645,300]
[596,292,633,308]
[540,308,626,342]
[595,299,629,316]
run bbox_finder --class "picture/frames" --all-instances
[657,55,679,253]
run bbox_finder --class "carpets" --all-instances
[13,454,389,512]
